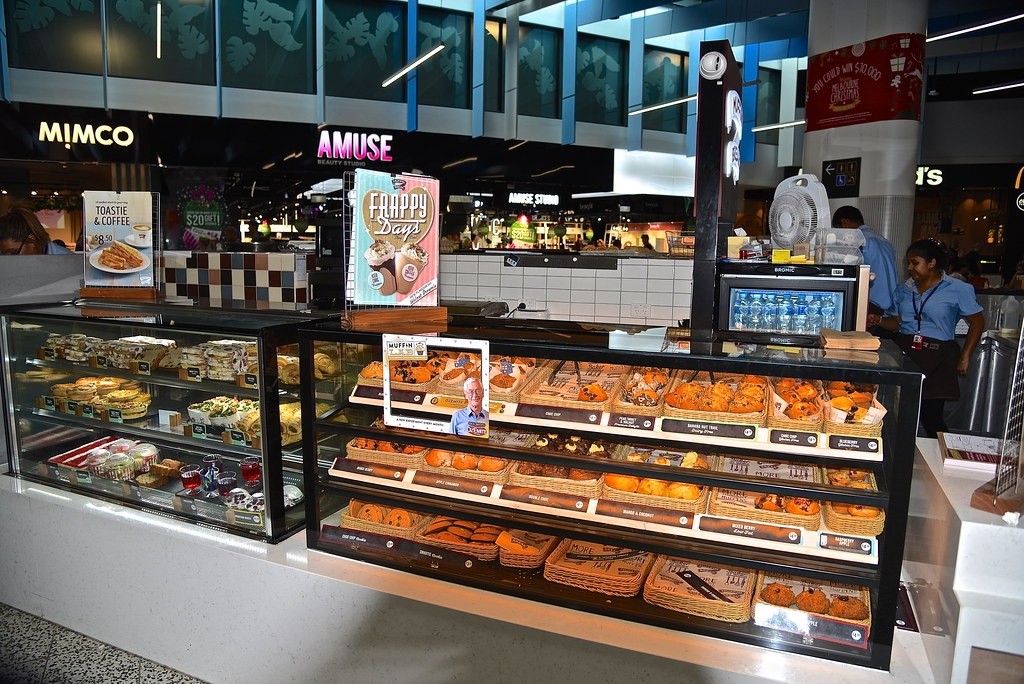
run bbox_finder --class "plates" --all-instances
[125,235,152,248]
[89,250,150,273]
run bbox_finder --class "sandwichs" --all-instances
[97,241,143,271]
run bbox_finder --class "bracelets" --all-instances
[878,314,882,325]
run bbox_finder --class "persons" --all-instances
[40,239,76,255]
[866,238,986,439]
[246,221,264,238]
[0,198,106,465]
[613,240,621,249]
[583,242,595,251]
[449,377,489,438]
[223,227,244,252]
[641,234,652,249]
[195,236,214,251]
[438,212,467,254]
[833,206,899,315]
[949,266,989,289]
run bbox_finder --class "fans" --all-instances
[768,173,831,250]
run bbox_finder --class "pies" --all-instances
[236,401,349,446]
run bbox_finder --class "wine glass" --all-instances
[199,467,219,498]
[240,457,261,487]
[217,471,237,502]
[179,464,200,495]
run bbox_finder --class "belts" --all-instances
[922,341,957,349]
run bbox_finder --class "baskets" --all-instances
[340,350,883,645]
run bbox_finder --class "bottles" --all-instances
[999,296,1024,333]
[733,292,835,335]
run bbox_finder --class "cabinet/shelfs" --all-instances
[6,321,896,661]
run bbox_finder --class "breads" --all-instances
[42,332,181,372]
[181,339,337,384]
[136,459,185,489]
[347,497,508,546]
[352,418,510,472]
[361,349,535,392]
[50,375,150,420]
[760,581,870,621]
[577,371,873,423]
[756,470,881,518]
[582,245,650,252]
[516,434,710,501]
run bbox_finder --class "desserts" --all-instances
[86,441,157,481]
[223,482,303,512]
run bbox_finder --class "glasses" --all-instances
[0,234,32,256]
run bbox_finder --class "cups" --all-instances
[132,224,151,245]
[202,454,223,472]
[396,249,424,294]
[364,245,396,295]
